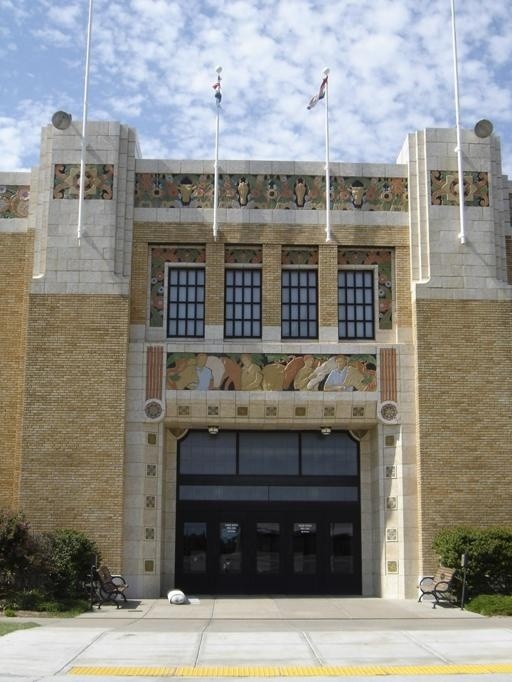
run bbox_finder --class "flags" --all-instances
[307,77,328,111]
[212,75,223,111]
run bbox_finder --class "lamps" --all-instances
[322,426,332,435]
[209,426,219,435]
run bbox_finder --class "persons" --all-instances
[167,353,377,392]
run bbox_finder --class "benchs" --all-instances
[416,563,461,610]
[90,566,131,610]
